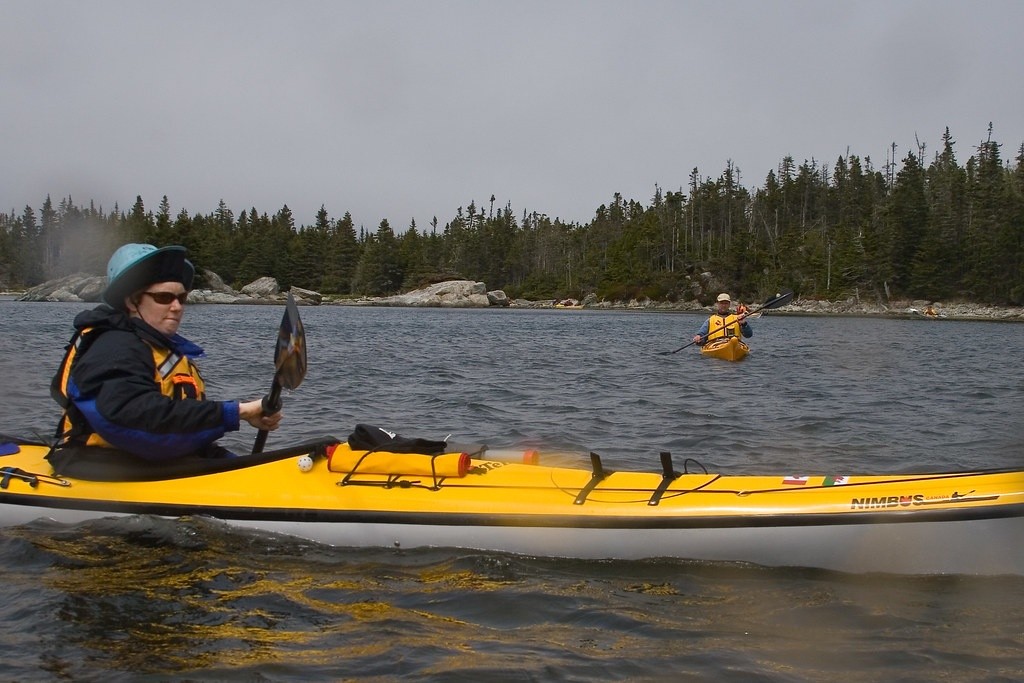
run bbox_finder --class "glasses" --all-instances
[142,291,187,305]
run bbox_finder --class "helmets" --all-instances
[102,244,186,309]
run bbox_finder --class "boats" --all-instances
[912,309,946,320]
[701,336,749,362]
[0,422,1024,578]
[556,303,585,309]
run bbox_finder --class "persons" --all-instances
[693,293,753,346]
[52,243,283,461]
[924,306,938,317]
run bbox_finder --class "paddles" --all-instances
[251,291,307,455]
[658,288,795,356]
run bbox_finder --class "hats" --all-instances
[717,293,731,302]
[121,256,193,297]
